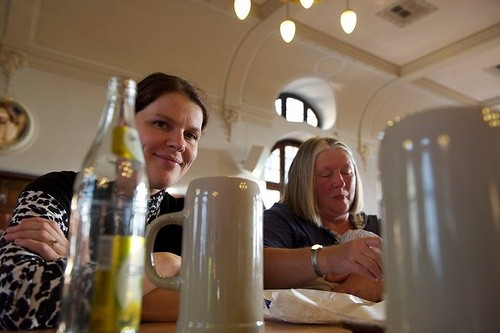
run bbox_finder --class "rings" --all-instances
[49,239,59,247]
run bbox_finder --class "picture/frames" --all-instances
[0,96,36,153]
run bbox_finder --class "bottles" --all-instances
[58,74,150,333]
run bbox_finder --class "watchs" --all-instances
[311,244,323,276]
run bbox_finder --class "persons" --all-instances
[0,72,209,333]
[261,135,385,303]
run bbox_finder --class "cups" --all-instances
[143,175,265,333]
[377,105,500,333]
